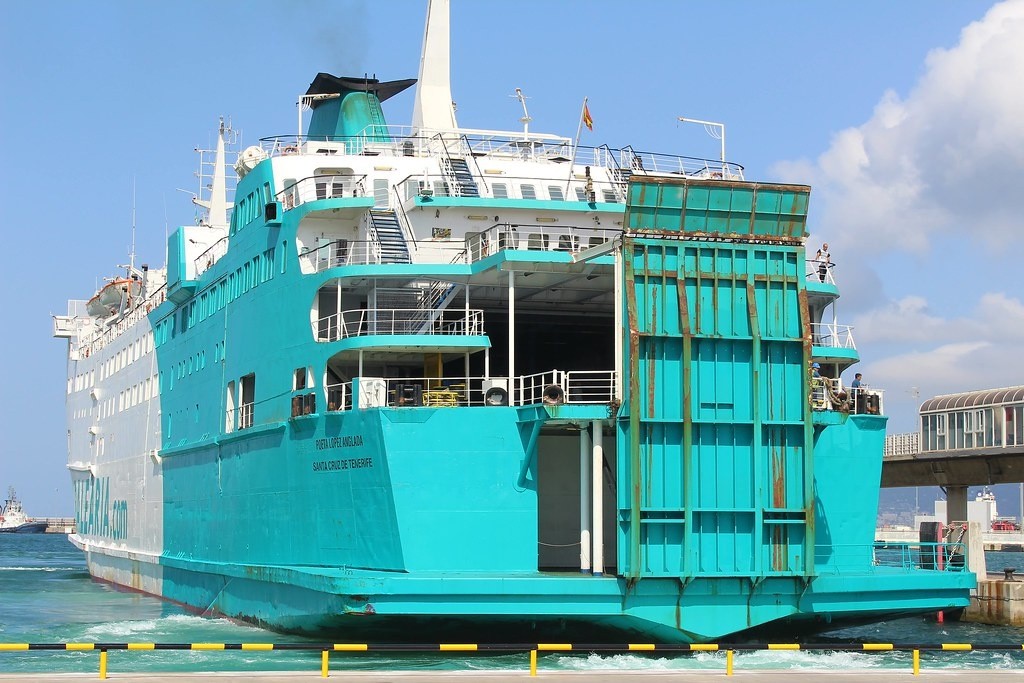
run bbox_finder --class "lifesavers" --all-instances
[281,145,298,156]
[544,385,563,404]
[485,387,508,405]
[710,171,726,180]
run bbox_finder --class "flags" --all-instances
[582,105,593,131]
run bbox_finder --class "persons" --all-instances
[814,242,830,282]
[810,362,822,405]
[848,373,865,401]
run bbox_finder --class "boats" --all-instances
[50,0,976,647]
[0,487,49,535]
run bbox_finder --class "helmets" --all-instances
[812,362,820,368]
